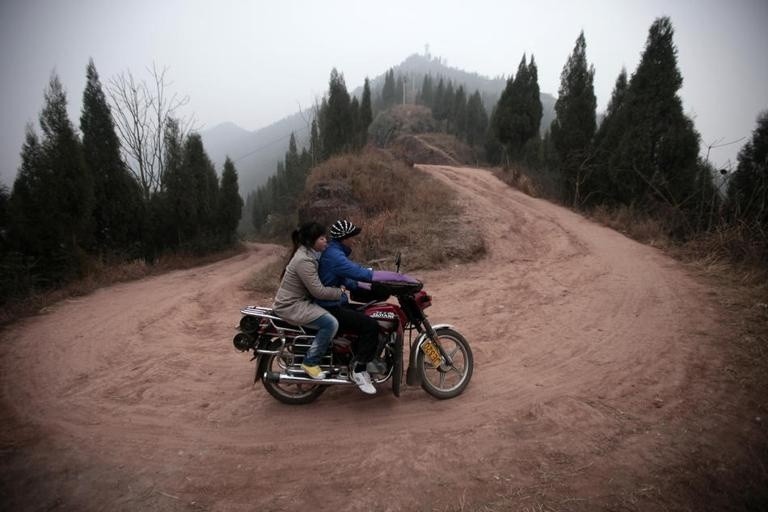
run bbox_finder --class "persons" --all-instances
[272,223,345,379]
[311,219,377,394]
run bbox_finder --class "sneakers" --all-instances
[350,368,377,395]
[301,363,326,380]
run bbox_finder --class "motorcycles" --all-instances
[233,251,474,405]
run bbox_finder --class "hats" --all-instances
[327,220,362,240]
